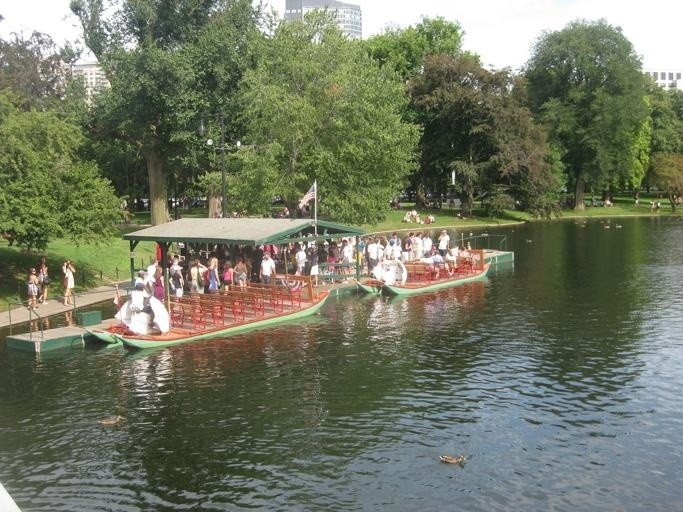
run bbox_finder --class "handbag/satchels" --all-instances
[43,276,52,285]
[197,270,210,287]
[239,272,249,281]
[172,270,183,289]
[62,276,68,289]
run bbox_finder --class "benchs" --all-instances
[397,256,473,283]
[160,279,301,332]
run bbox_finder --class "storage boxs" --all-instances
[75,309,101,327]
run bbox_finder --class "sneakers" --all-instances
[42,301,48,305]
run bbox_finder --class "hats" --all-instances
[138,270,145,275]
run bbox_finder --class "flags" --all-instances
[114,290,121,307]
[296,182,315,210]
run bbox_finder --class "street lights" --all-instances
[204,116,241,217]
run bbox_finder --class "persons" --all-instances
[591,193,683,213]
[61,259,76,306]
[65,311,74,326]
[391,195,469,225]
[271,206,291,219]
[31,318,49,332]
[25,256,48,312]
